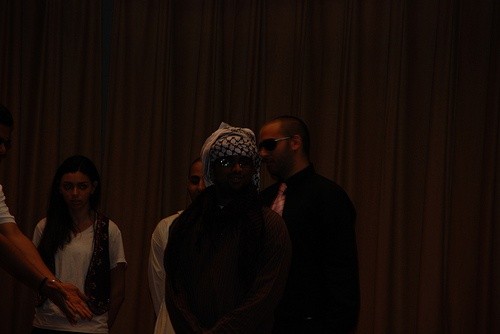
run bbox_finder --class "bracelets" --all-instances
[40,277,48,296]
[51,279,61,284]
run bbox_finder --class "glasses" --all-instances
[258,137,290,153]
[211,155,254,170]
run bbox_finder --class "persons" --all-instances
[163,122,292,334]
[32,154,127,334]
[259,116,361,334]
[0,101,93,326]
[147,156,207,334]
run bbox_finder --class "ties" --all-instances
[271,183,288,217]
